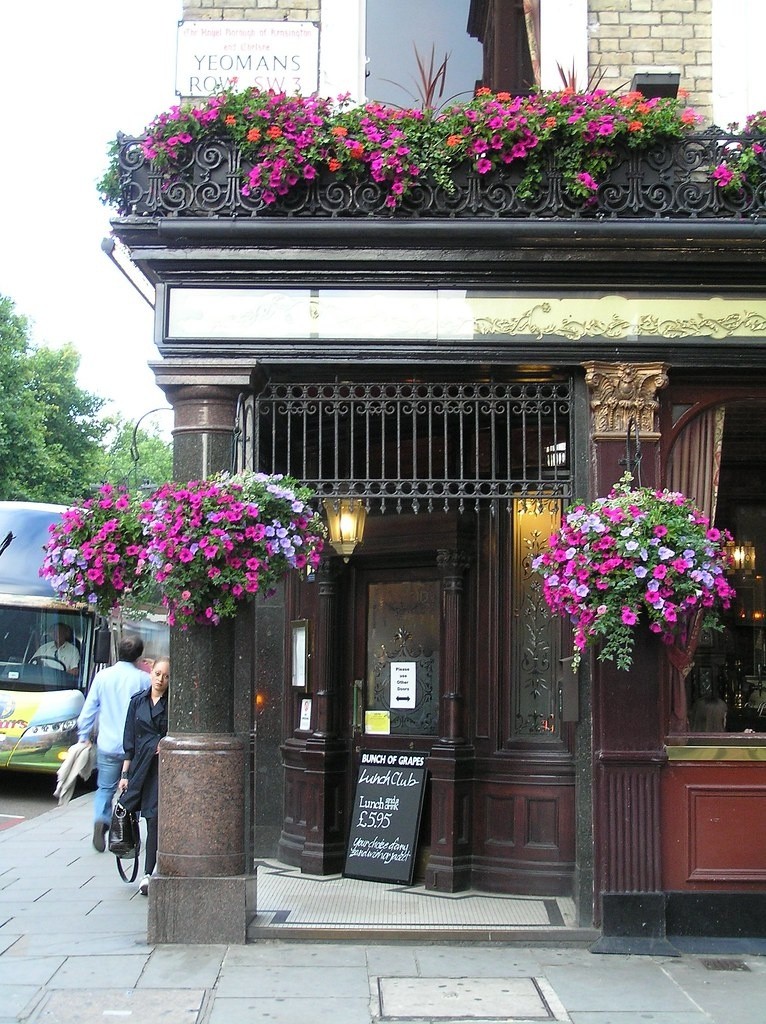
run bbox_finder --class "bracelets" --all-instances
[121,771,128,779]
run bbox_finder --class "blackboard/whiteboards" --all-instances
[338,748,431,885]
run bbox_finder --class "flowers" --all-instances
[529,473,734,672]
[37,466,327,630]
[94,84,766,210]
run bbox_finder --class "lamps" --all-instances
[321,472,366,564]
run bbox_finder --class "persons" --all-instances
[75,634,152,852]
[115,656,170,895]
[28,623,81,678]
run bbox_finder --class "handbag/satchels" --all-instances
[108,787,141,884]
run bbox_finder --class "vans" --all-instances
[0,593,111,790]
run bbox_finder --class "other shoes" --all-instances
[92,818,107,854]
[139,873,150,896]
